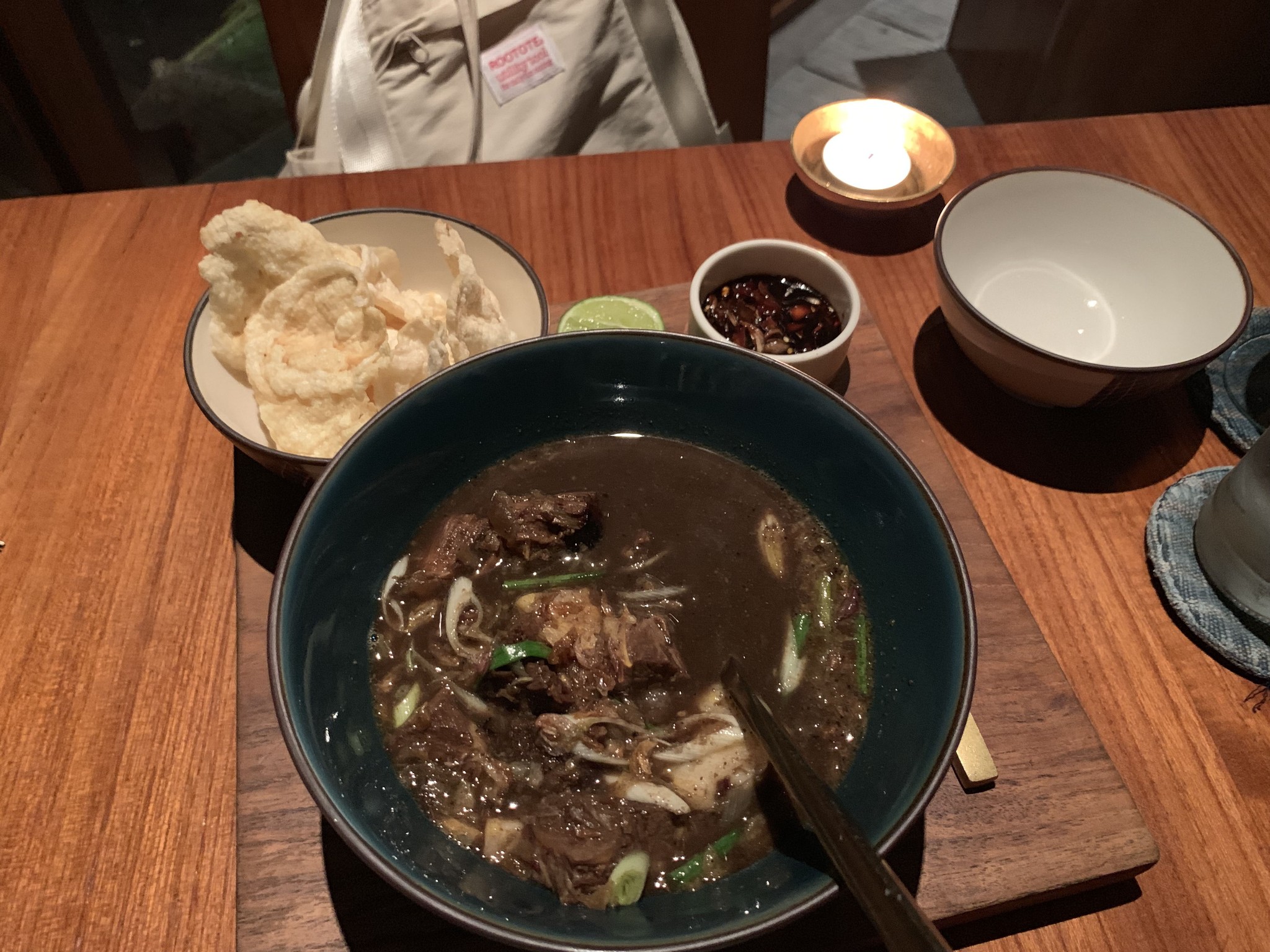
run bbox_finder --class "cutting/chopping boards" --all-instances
[235,284,1159,951]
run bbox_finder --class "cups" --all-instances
[1193,424,1270,627]
[686,238,860,387]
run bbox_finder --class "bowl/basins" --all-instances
[932,166,1254,409]
[789,98,956,222]
[182,210,550,492]
[263,332,978,951]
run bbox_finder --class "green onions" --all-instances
[382,515,868,905]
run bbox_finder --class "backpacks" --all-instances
[286,0,735,178]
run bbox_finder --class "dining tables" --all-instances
[0,101,1270,952]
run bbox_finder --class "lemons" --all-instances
[557,295,665,333]
